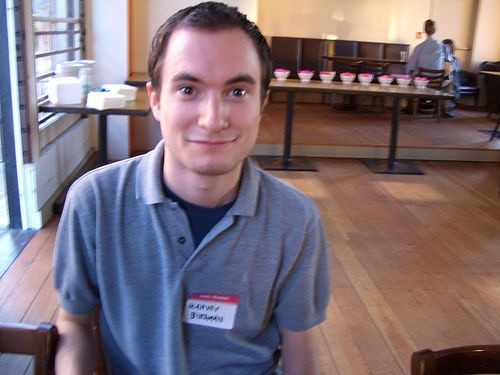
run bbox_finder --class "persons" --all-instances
[442,39,460,111]
[54,1,331,375]
[406,19,455,117]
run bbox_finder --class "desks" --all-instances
[39,87,150,166]
[126,72,456,176]
[323,55,407,112]
[480,71,500,141]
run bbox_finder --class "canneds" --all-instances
[79,68,95,98]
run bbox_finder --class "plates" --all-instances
[47,59,96,104]
[102,84,138,101]
[86,91,126,112]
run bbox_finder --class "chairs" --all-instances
[409,67,445,122]
[456,70,479,107]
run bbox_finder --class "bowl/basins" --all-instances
[396,78,412,86]
[298,73,314,81]
[340,76,356,82]
[358,76,374,83]
[414,80,430,88]
[274,71,291,80]
[319,74,336,82]
[378,77,394,85]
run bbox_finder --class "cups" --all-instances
[79,68,95,97]
